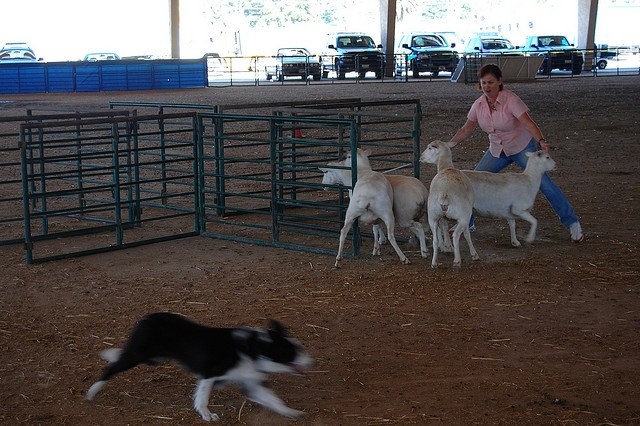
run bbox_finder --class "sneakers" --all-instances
[571,221,585,244]
[449,223,476,233]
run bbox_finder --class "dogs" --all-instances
[84,310,319,424]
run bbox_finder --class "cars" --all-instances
[394,33,460,77]
[519,36,583,75]
[463,32,519,58]
[0,42,43,62]
[264,47,323,81]
[320,33,386,80]
[84,52,121,62]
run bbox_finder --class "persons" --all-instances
[448,62,584,242]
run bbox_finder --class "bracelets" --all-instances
[537,138,545,146]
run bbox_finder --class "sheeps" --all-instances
[419,138,481,270]
[318,161,430,260]
[459,149,560,248]
[335,147,414,269]
